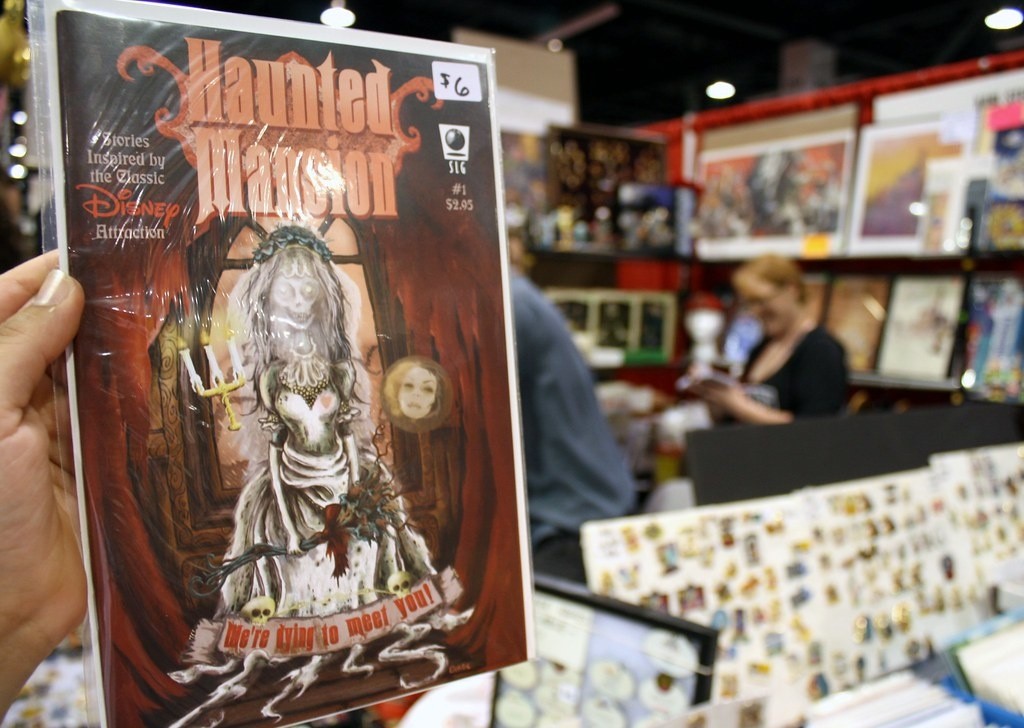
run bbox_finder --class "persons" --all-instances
[508,263,641,584]
[683,254,848,426]
